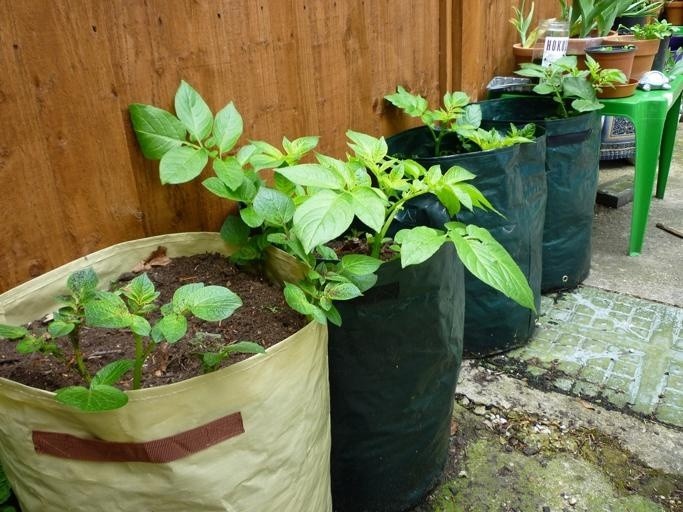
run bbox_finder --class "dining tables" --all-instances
[492,0,683,97]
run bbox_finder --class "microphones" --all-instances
[590,75,682,260]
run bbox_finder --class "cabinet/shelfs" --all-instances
[528,20,570,85]
